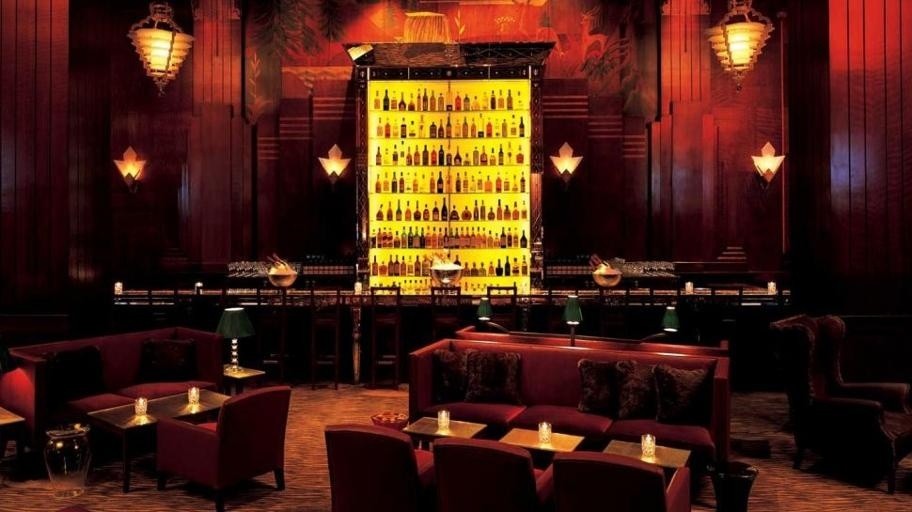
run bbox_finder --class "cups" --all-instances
[133,398,148,416]
[194,281,205,295]
[113,281,126,294]
[640,433,657,457]
[437,410,451,429]
[42,423,92,498]
[684,281,694,294]
[537,422,554,444]
[767,281,778,295]
[187,387,200,405]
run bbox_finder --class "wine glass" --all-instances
[226,259,302,277]
[591,269,625,301]
[267,271,298,298]
[614,259,676,278]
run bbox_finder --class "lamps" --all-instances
[704,0,776,94]
[549,140,584,185]
[751,141,786,190]
[126,2,196,97]
[317,143,351,185]
[112,144,147,194]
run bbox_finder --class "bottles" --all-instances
[536,250,596,276]
[369,83,530,291]
[299,254,355,276]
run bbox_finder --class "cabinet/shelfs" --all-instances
[351,63,546,290]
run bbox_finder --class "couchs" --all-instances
[0,325,224,431]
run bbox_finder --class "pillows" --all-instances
[37,344,107,414]
[138,336,195,382]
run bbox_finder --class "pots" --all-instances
[429,265,463,294]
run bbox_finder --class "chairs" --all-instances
[152,383,292,511]
[768,313,912,495]
